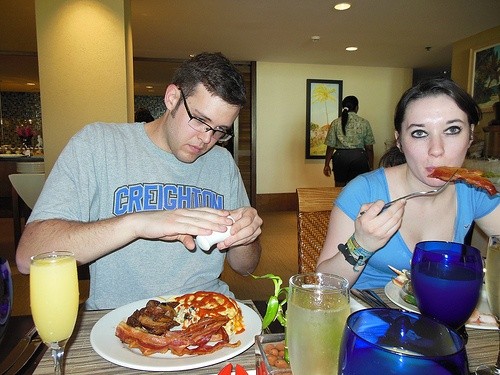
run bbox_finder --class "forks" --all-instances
[359,169,459,215]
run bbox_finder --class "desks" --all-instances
[8,174,45,253]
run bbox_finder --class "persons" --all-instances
[323,95,375,187]
[16,51,263,309]
[315,77,500,290]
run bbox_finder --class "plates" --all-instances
[16,162,44,174]
[384,274,500,330]
[89,294,263,372]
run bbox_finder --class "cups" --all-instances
[410,240,484,333]
[337,307,471,375]
[286,271,351,375]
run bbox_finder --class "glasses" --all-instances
[177,87,235,142]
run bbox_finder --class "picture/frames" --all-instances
[305,79,343,159]
[467,39,500,112]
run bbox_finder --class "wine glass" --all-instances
[29,250,79,375]
[475,235,500,375]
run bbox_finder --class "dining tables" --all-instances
[0,288,500,375]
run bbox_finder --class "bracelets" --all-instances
[337,234,374,271]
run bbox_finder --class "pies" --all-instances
[175,291,243,334]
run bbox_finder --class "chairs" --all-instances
[297,186,343,283]
